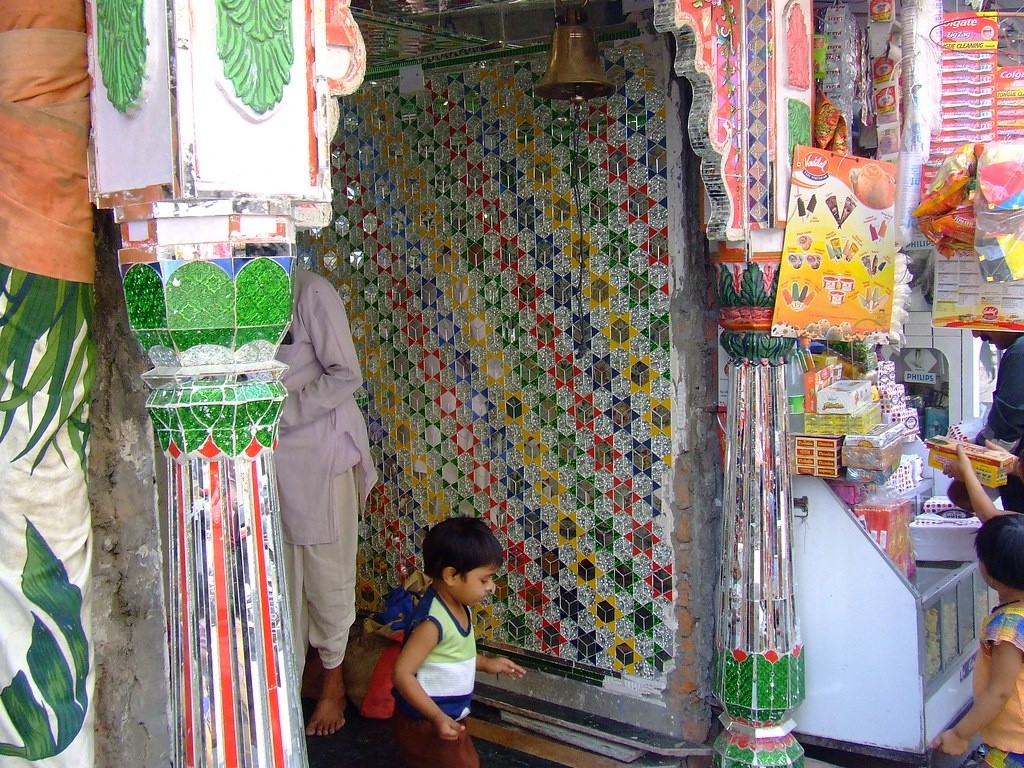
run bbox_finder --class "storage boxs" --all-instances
[795,340,1015,562]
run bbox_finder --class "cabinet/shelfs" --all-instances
[790,474,991,756]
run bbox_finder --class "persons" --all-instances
[272,267,380,739]
[391,516,528,768]
[942,438,1024,524]
[947,328,1024,513]
[926,513,1024,768]
